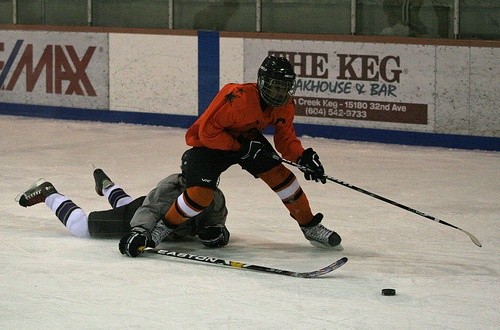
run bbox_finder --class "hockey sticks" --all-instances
[272,155,483,248]
[138,246,349,278]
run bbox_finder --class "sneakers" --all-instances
[93,167,111,197]
[145,220,174,250]
[14,177,56,207]
[299,213,344,253]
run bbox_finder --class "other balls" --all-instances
[381,289,396,296]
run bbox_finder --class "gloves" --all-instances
[119,231,148,258]
[299,147,326,184]
[238,139,268,167]
[197,224,227,249]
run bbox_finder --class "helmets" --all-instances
[257,55,295,107]
[181,149,191,177]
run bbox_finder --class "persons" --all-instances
[14,147,231,258]
[145,55,344,251]
